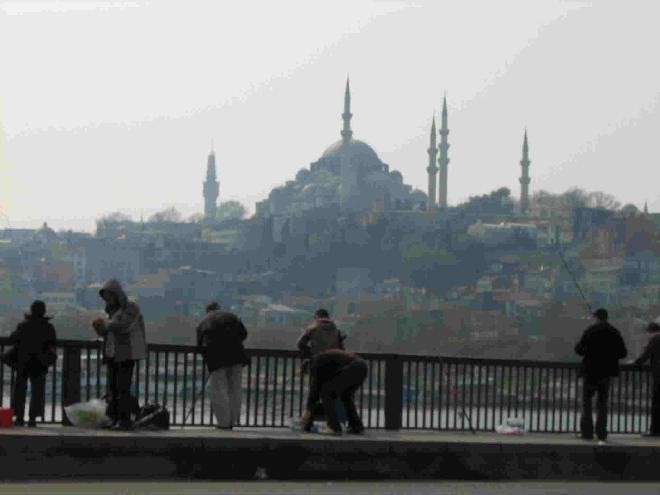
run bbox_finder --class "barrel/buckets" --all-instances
[507,417,524,430]
[0,408,14,426]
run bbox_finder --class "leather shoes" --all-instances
[214,424,238,431]
[330,429,360,436]
[576,432,607,441]
[641,431,659,438]
[13,418,36,427]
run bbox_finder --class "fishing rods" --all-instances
[430,351,475,433]
[541,228,593,317]
[180,372,214,429]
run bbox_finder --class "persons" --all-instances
[573,307,627,441]
[3,300,58,429]
[297,309,370,436]
[626,322,660,439]
[92,279,150,430]
[196,302,249,430]
